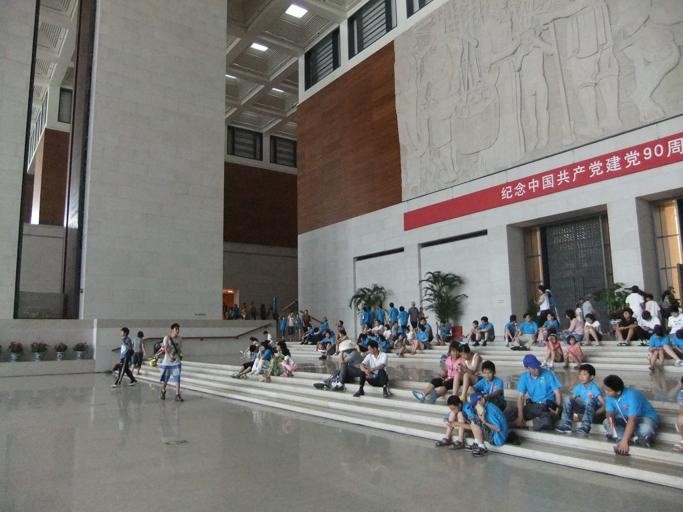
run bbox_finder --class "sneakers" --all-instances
[575,425,590,435]
[112,382,121,388]
[464,441,479,451]
[472,445,489,457]
[555,421,572,434]
[447,440,465,450]
[424,391,437,403]
[160,390,166,400]
[127,379,136,386]
[434,438,451,447]
[175,395,183,401]
[412,390,425,402]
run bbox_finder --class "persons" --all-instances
[412,341,465,403]
[545,289,556,315]
[645,294,662,320]
[378,335,388,352]
[435,320,448,346]
[411,324,427,355]
[393,333,406,358]
[392,321,399,340]
[386,302,400,327]
[534,285,550,326]
[602,374,660,455]
[472,316,495,346]
[575,302,583,321]
[663,328,683,366]
[562,334,586,370]
[358,326,369,352]
[537,312,559,347]
[360,307,369,334]
[463,392,510,456]
[668,307,683,334]
[561,309,583,345]
[375,304,385,325]
[130,330,146,375]
[368,306,375,328]
[312,340,362,390]
[510,313,538,350]
[554,364,605,436]
[579,297,595,319]
[466,361,507,412]
[300,301,349,360]
[397,306,409,329]
[371,319,384,336]
[616,308,640,347]
[220,300,301,342]
[353,340,390,398]
[504,314,518,346]
[452,343,483,401]
[583,313,604,346]
[668,375,683,452]
[625,285,645,318]
[383,323,394,347]
[435,395,476,449]
[504,354,563,430]
[647,325,670,370]
[159,323,183,401]
[636,311,661,345]
[465,320,481,342]
[408,301,419,328]
[540,333,564,369]
[403,325,414,346]
[111,327,138,388]
[232,331,298,384]
[414,318,434,342]
[367,332,379,342]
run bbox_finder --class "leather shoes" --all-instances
[353,390,364,397]
[382,388,388,397]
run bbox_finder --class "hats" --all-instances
[523,354,541,369]
[466,392,483,408]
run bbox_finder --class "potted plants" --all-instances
[73,343,87,360]
[8,342,23,360]
[31,342,48,361]
[55,344,68,361]
[593,282,629,325]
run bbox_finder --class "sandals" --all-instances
[672,439,683,452]
